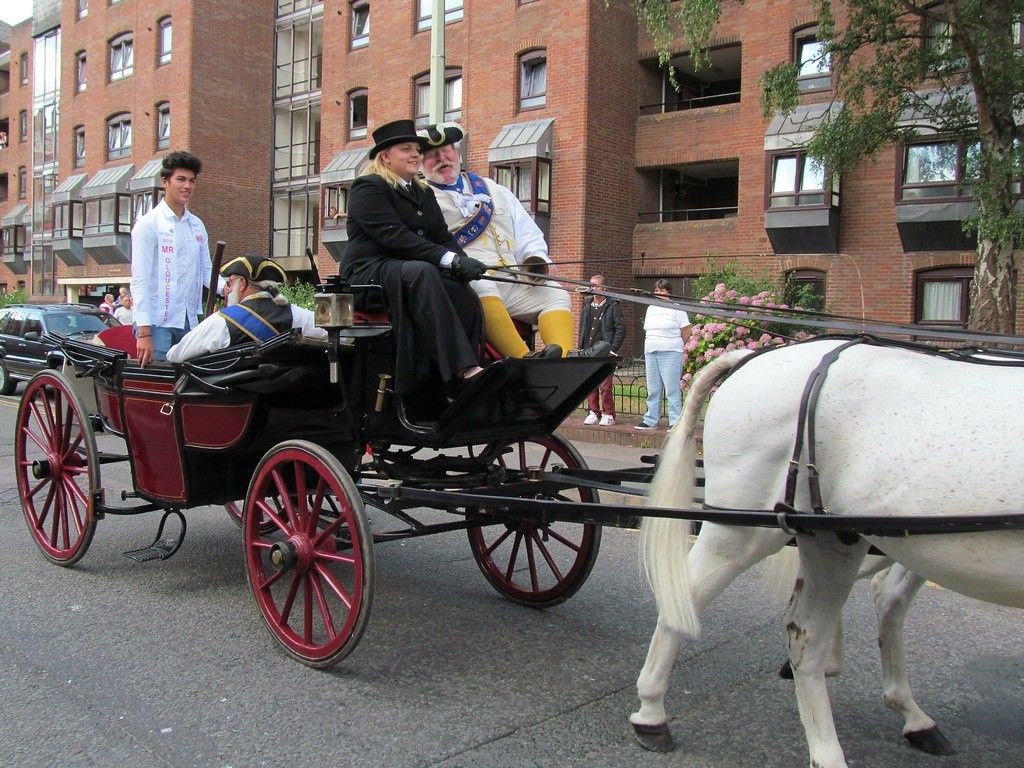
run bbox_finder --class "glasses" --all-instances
[226,276,240,287]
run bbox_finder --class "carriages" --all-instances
[13,274,1024,767]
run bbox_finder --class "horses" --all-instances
[628,337,1024,768]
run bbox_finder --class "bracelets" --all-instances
[137,334,152,339]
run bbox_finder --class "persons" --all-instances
[578,275,627,426]
[329,206,347,219]
[99,287,133,326]
[131,151,230,368]
[339,120,487,420]
[416,121,612,359]
[635,278,692,433]
[166,253,329,363]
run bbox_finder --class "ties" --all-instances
[406,185,417,198]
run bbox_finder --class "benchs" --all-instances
[98,322,368,394]
[314,283,535,343]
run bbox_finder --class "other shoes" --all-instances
[567,341,612,358]
[522,344,562,359]
[667,425,674,432]
[634,423,658,429]
[447,359,501,402]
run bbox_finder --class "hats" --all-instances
[219,254,287,283]
[417,121,466,154]
[369,120,430,160]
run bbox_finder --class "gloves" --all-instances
[521,258,548,284]
[452,254,486,281]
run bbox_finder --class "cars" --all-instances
[0,301,124,396]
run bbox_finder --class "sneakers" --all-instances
[599,414,616,425]
[584,411,599,424]
